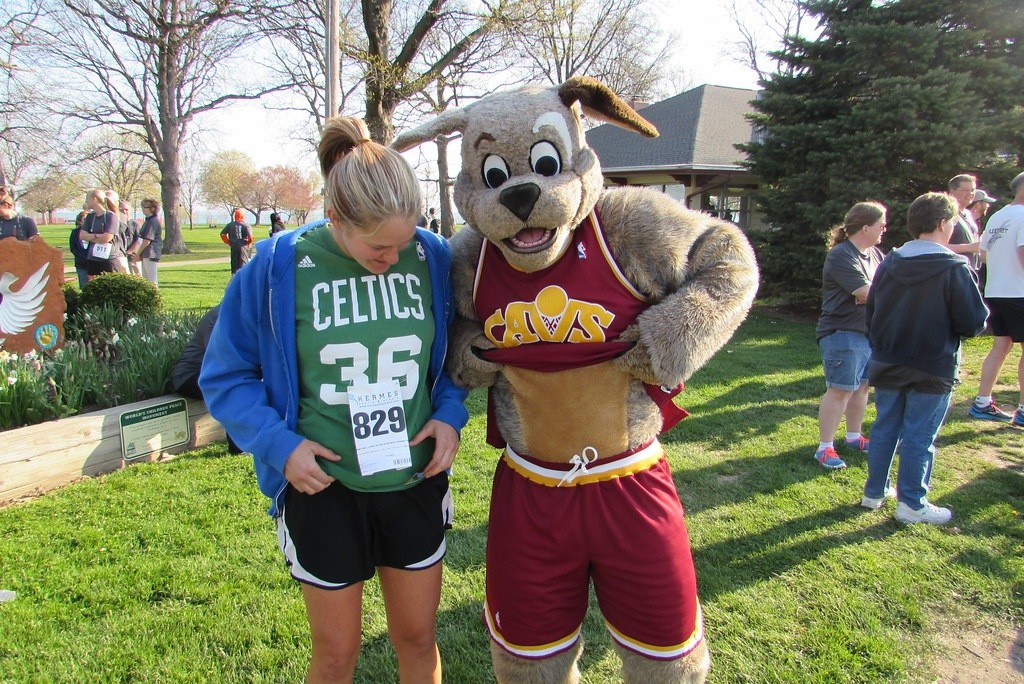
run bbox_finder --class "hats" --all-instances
[973,190,997,202]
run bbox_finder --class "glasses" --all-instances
[868,223,885,231]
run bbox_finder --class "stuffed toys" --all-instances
[390,79,758,684]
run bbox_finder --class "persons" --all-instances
[814,202,886,467]
[711,205,732,220]
[198,116,468,684]
[969,172,1024,424]
[947,173,995,269]
[862,193,990,524]
[0,186,38,240]
[220,211,254,275]
[270,212,285,237]
[417,208,440,233]
[69,189,162,290]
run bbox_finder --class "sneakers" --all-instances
[861,485,897,510]
[844,435,869,453]
[969,399,1013,422]
[894,496,952,526]
[1013,404,1024,425]
[814,447,846,469]
